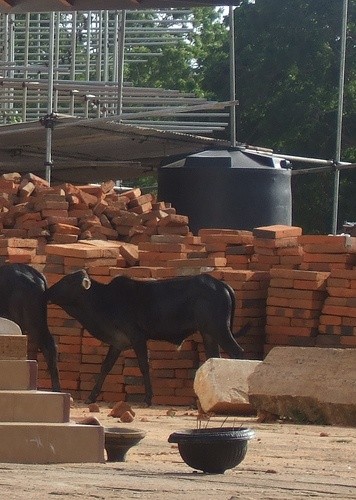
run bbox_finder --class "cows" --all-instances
[0,262,62,393]
[46,270,244,410]
